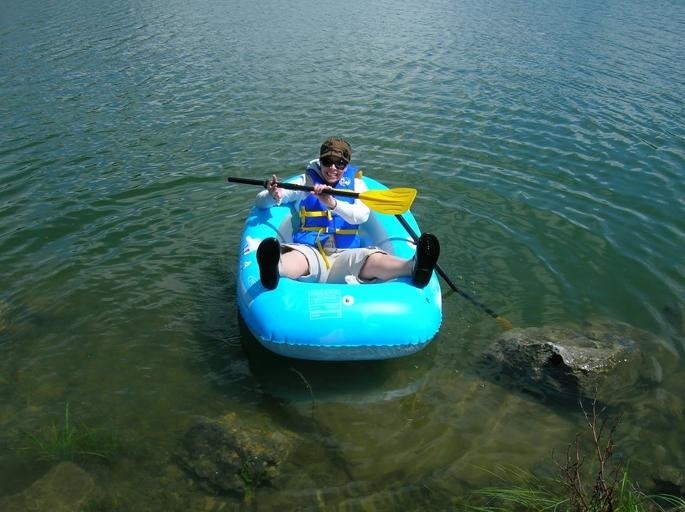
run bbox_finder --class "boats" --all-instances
[236,173,442,362]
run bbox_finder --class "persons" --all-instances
[255,138,441,290]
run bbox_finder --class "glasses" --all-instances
[320,156,348,170]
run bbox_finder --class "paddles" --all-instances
[228,177,417,215]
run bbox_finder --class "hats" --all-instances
[319,138,353,165]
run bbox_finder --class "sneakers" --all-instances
[256,236,281,289]
[411,231,442,288]
